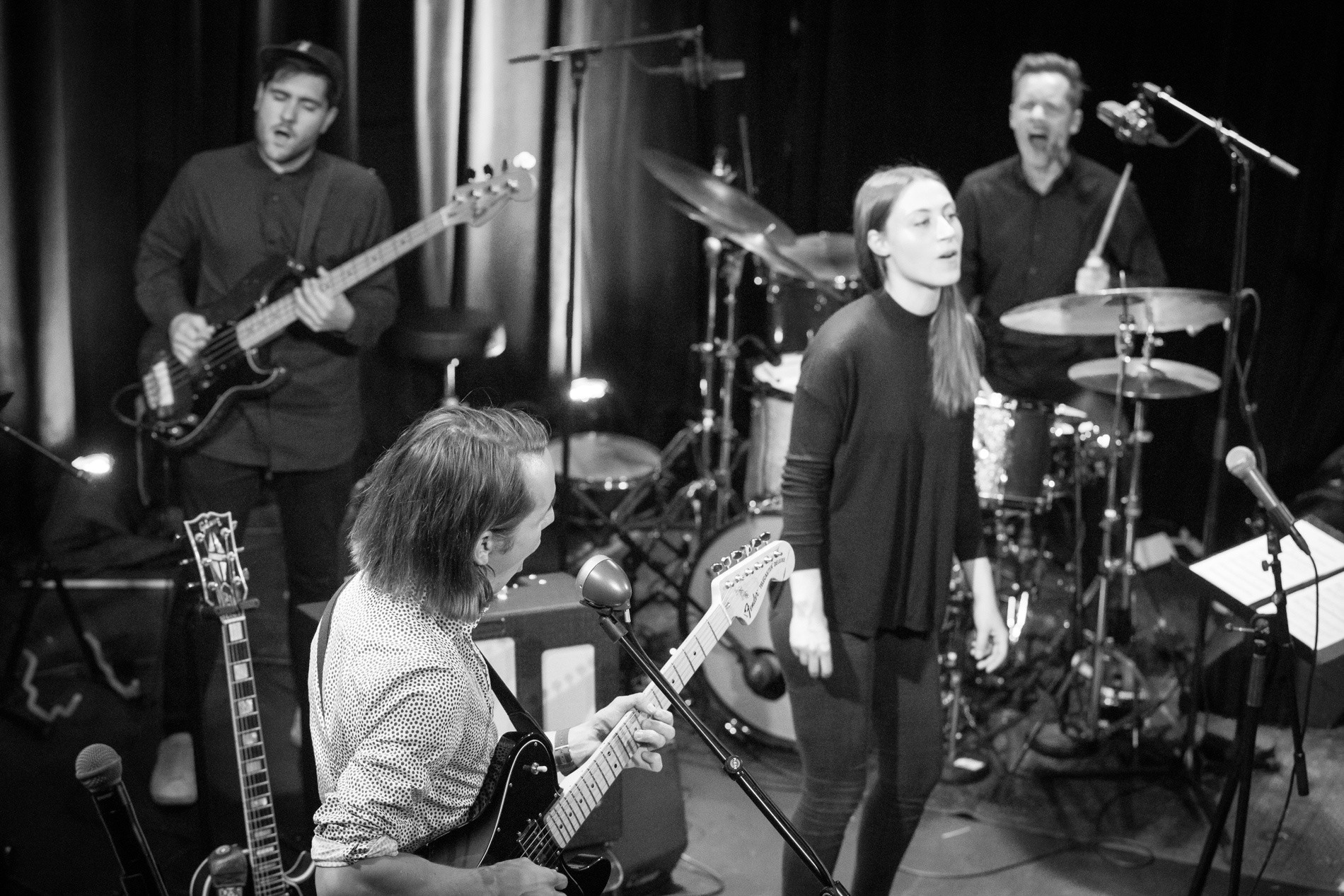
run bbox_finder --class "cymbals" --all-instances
[635,145,797,249]
[1069,355,1219,398]
[1000,287,1244,337]
[666,196,847,306]
[546,431,660,480]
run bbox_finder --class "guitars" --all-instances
[171,509,319,896]
[134,149,545,448]
[442,526,796,896]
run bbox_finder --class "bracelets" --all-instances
[553,727,578,777]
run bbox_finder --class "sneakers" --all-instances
[149,732,198,806]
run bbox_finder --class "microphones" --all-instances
[1185,289,1250,337]
[75,744,170,896]
[742,653,783,693]
[1096,100,1168,147]
[575,554,632,613]
[1225,445,1310,555]
[653,59,744,83]
[752,339,781,366]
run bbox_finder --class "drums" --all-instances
[1041,407,1105,487]
[966,392,1057,515]
[746,360,803,498]
[768,234,874,352]
[682,506,818,753]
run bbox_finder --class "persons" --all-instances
[307,405,676,896]
[956,53,1167,645]
[769,166,1011,895]
[133,40,399,805]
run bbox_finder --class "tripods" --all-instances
[606,229,747,613]
[968,303,1176,773]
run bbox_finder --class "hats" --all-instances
[258,41,343,106]
